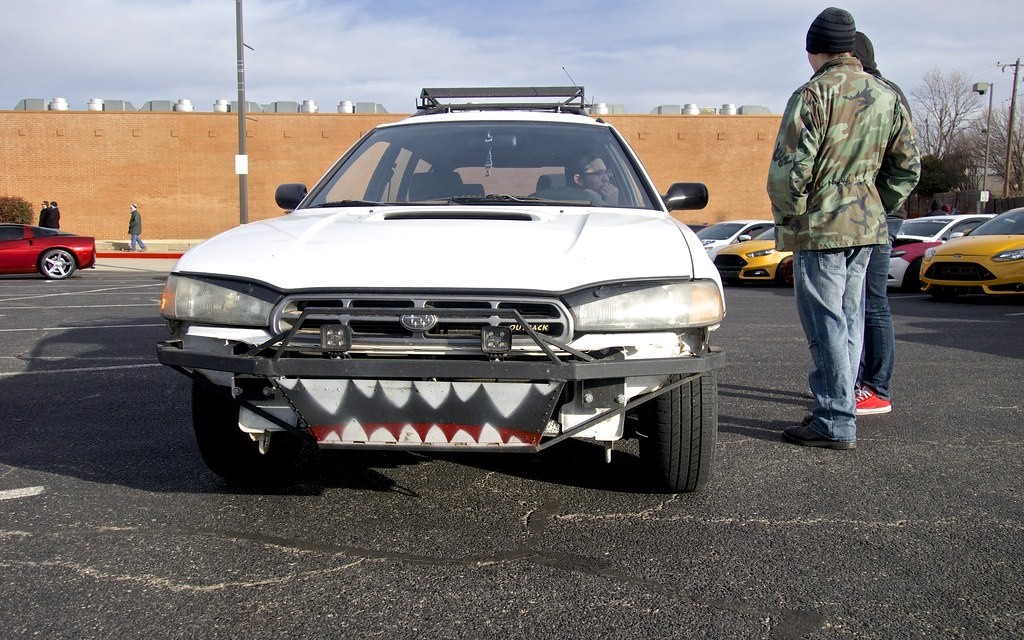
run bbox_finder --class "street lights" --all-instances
[971,82,996,213]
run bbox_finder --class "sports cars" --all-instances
[0,223,97,280]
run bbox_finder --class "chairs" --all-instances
[463,184,485,197]
[535,174,567,192]
[409,172,465,200]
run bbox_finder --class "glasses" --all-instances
[582,169,613,179]
[42,204,46,206]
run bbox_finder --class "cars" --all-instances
[884,206,1024,306]
[715,225,795,289]
[694,218,777,262]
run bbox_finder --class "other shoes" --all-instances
[128,250,136,252]
[140,247,146,252]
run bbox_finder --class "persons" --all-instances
[767,7,922,447]
[128,203,146,252]
[39,201,60,229]
[851,30,913,416]
[563,140,619,207]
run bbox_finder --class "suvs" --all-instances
[153,84,725,495]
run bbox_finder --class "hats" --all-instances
[131,203,138,213]
[806,7,857,54]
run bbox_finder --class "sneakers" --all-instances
[782,425,856,450]
[808,382,859,399]
[803,414,814,425]
[854,383,893,415]
